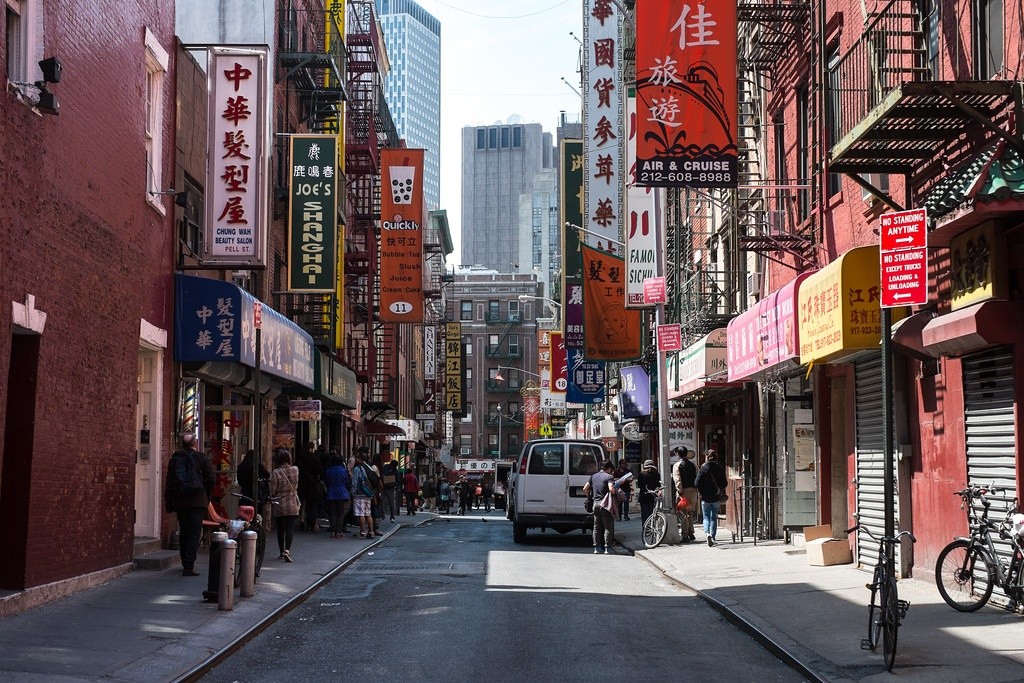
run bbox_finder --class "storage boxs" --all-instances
[803,524,852,566]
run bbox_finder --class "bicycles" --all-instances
[932,484,1023,613]
[641,483,668,549]
[844,525,920,671]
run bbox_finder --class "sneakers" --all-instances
[367,531,375,539]
[352,532,367,539]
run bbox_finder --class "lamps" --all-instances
[34,56,62,117]
[149,190,189,210]
[182,381,195,437]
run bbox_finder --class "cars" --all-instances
[230,490,284,586]
[511,438,610,543]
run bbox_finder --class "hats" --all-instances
[644,459,657,470]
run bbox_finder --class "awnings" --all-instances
[368,420,407,437]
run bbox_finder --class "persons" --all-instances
[164,435,504,577]
[582,446,728,555]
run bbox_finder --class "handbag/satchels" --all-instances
[296,496,301,510]
[716,487,728,503]
[584,499,594,513]
[314,480,327,497]
[676,496,692,512]
[360,470,381,498]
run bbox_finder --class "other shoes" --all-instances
[279,553,284,559]
[441,495,449,501]
[283,551,292,561]
[390,518,395,523]
[373,530,383,536]
[680,535,695,543]
[182,569,200,576]
[604,548,615,554]
[708,536,713,547]
[594,547,604,554]
